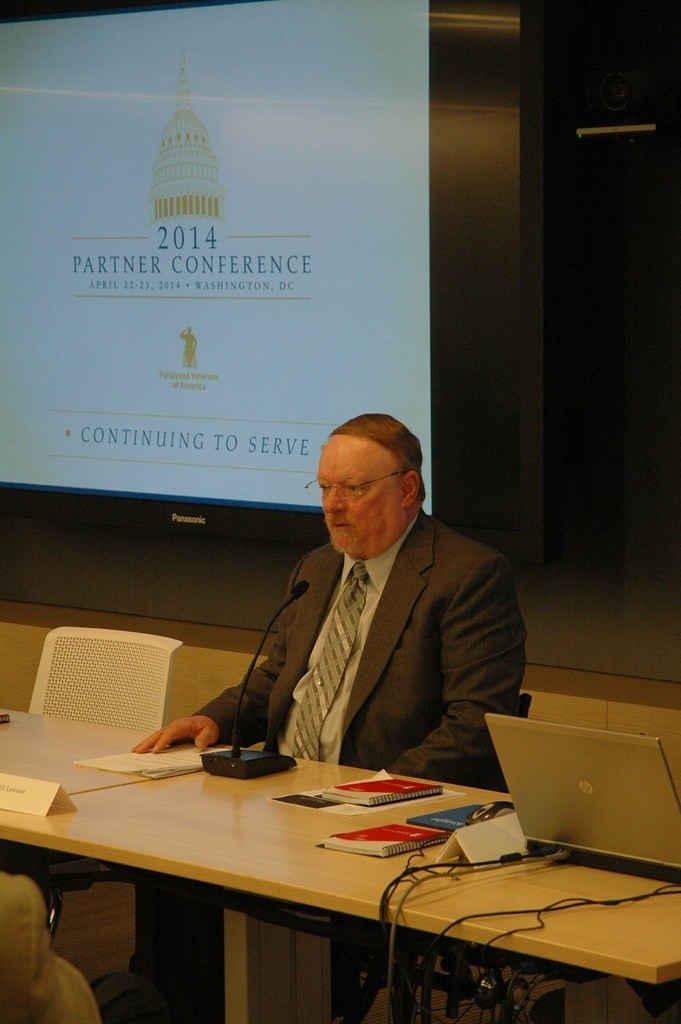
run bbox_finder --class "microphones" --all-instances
[199,579,310,778]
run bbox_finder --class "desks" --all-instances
[1,703,681,1023]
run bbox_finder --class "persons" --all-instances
[128,410,527,1024]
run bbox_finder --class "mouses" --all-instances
[464,801,514,827]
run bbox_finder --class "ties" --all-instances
[292,561,369,762]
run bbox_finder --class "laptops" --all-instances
[484,712,681,884]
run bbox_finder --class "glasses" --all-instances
[305,467,414,498]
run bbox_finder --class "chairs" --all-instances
[20,625,182,929]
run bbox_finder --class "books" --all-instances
[406,805,484,831]
[321,778,443,807]
[323,824,451,857]
